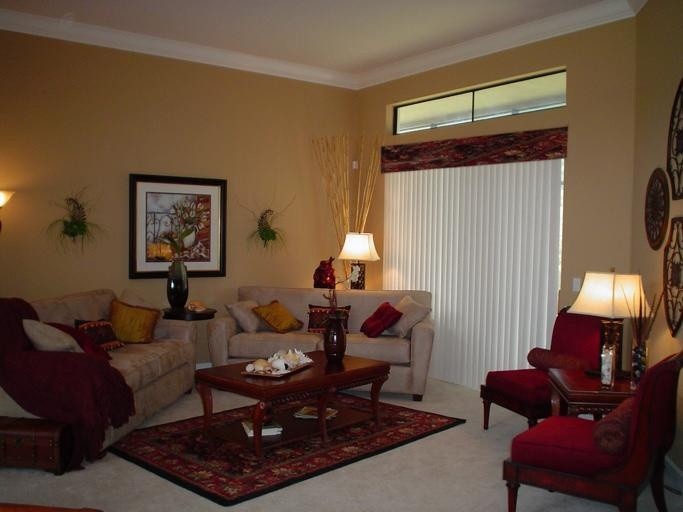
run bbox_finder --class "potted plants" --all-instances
[127,173,227,279]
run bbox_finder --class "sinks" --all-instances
[74,319,123,350]
[0,288,195,451]
[208,286,434,401]
[21,320,82,352]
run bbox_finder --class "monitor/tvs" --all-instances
[293,406,338,420]
[241,420,283,437]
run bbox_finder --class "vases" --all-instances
[321,265,360,315]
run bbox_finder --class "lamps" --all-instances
[480,305,605,431]
[502,352,681,512]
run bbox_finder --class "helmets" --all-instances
[240,362,312,377]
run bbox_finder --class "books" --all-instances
[1,416,71,471]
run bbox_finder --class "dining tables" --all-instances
[620,286,665,389]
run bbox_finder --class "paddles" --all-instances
[111,392,467,507]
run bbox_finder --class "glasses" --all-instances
[566,271,655,389]
[0,189,15,230]
[337,232,380,290]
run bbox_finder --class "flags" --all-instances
[324,315,347,362]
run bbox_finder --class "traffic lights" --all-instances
[111,299,162,343]
[386,295,432,337]
[252,299,303,334]
[359,301,403,338]
[307,304,351,333]
[224,300,260,335]
